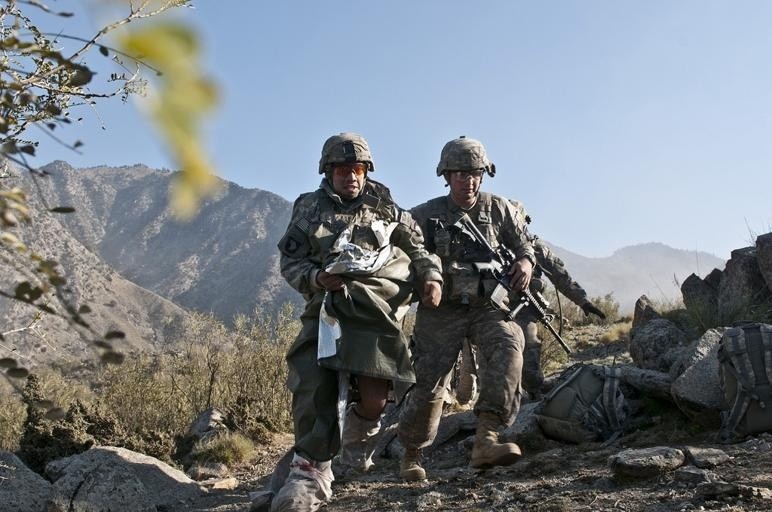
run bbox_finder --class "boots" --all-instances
[399,445,427,480]
[470,412,521,471]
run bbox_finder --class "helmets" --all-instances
[318,132,374,172]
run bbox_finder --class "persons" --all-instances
[455,198,607,405]
[270,133,445,512]
[397,135,537,482]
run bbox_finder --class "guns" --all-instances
[444,213,572,355]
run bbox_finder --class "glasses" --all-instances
[331,163,368,175]
[446,169,487,180]
[436,137,496,178]
